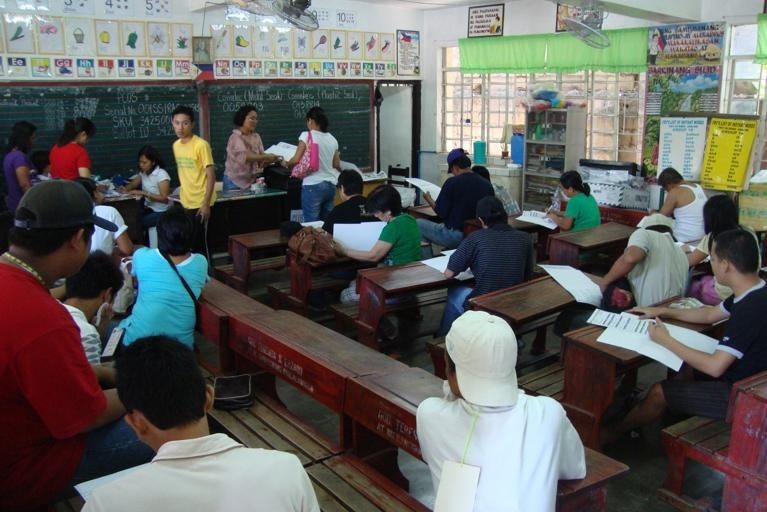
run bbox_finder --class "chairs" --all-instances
[388,165,410,190]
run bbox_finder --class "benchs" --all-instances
[194,352,343,467]
[426,312,561,380]
[656,371,765,509]
[331,288,448,335]
[213,256,288,284]
[517,361,576,399]
[267,272,348,310]
[300,453,430,512]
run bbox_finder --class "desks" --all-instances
[356,254,475,350]
[561,296,729,451]
[104,195,147,247]
[167,188,290,256]
[344,367,631,511]
[549,221,637,271]
[228,309,408,449]
[330,178,390,201]
[408,204,438,222]
[286,241,429,302]
[469,269,606,356]
[721,379,767,511]
[230,229,290,278]
[463,215,562,264]
[194,278,275,372]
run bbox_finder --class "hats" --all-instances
[446,148,468,173]
[14,178,120,232]
[445,310,519,409]
[636,213,680,244]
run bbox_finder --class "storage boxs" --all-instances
[577,157,651,211]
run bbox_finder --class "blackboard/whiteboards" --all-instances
[1,78,376,195]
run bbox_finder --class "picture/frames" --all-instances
[554,3,604,33]
[0,13,421,81]
[465,2,505,39]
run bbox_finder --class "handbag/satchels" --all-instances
[600,277,636,314]
[262,154,298,190]
[290,129,319,179]
[211,374,256,412]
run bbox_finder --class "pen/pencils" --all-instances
[653,322,660,326]
[548,202,554,213]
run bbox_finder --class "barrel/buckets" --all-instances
[509,132,524,165]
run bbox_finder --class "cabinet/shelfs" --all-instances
[519,101,587,212]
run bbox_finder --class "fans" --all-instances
[563,2,611,51]
[272,1,321,35]
